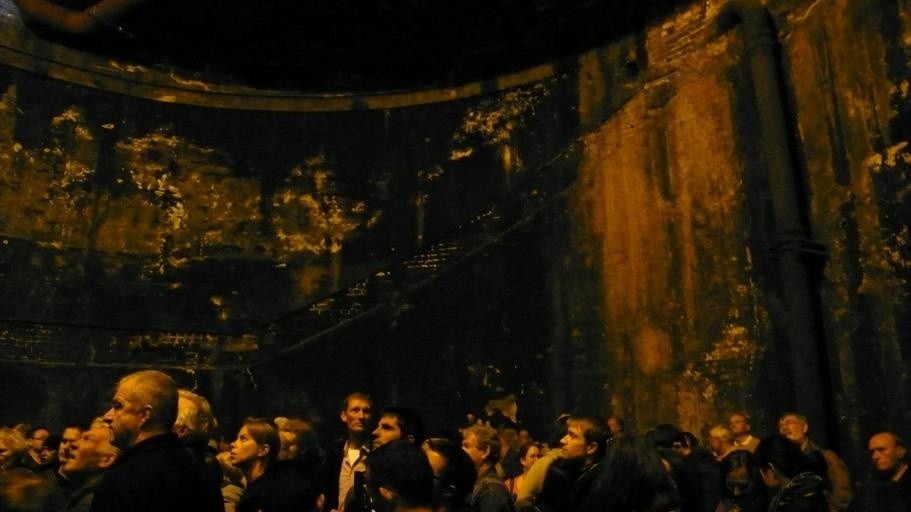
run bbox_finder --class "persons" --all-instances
[0,368,910,512]
